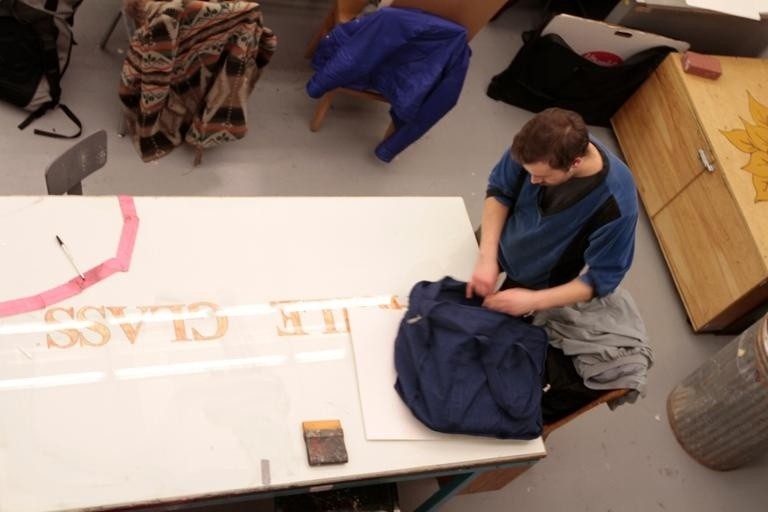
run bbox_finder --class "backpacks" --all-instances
[0,0,84,113]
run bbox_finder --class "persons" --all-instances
[466,108,638,317]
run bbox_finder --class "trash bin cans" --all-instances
[667,309,768,472]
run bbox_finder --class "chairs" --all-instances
[433,342,629,493]
[101,0,259,138]
[303,0,508,143]
[45,129,107,196]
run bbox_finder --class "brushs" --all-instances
[302,419,348,466]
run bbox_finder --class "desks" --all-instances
[1,196,546,512]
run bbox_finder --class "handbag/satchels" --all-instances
[393,276,547,440]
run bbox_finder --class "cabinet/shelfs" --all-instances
[610,55,767,332]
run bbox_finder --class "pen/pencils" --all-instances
[56,235,86,282]
